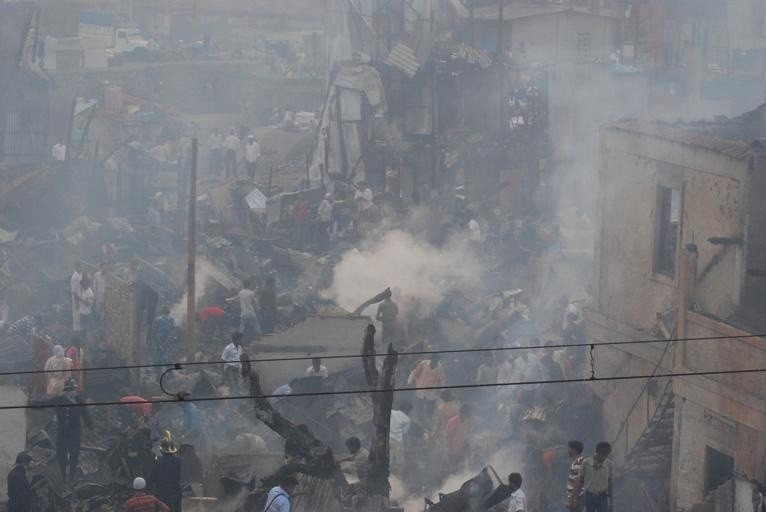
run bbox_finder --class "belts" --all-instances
[586,491,610,497]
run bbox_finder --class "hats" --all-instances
[160,431,177,453]
[63,377,78,391]
[133,477,146,490]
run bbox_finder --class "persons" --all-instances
[0,113,615,511]
